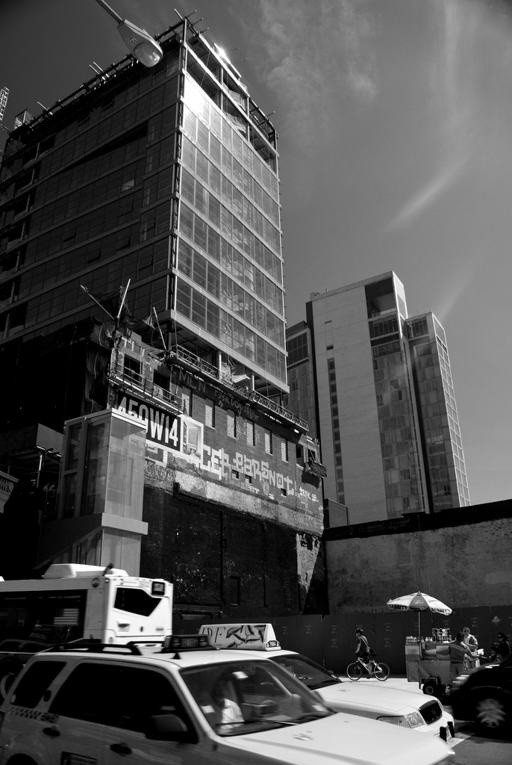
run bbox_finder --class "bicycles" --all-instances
[346,653,390,681]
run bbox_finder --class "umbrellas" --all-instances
[384,590,453,689]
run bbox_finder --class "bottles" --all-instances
[406,627,452,644]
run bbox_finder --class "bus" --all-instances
[0,563,175,655]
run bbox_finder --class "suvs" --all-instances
[1,638,456,764]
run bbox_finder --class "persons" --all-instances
[462,627,479,651]
[353,628,371,662]
[490,632,510,663]
[446,633,477,683]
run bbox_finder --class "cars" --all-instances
[450,659,511,738]
[196,623,456,743]
